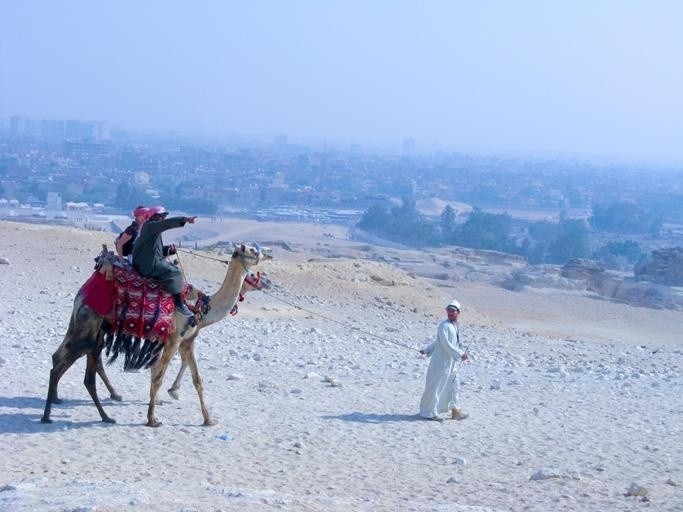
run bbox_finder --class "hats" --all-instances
[134,206,169,219]
[446,301,460,312]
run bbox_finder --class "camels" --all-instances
[39,241,274,427]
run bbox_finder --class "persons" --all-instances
[419,299,470,422]
[114,207,148,266]
[132,205,194,316]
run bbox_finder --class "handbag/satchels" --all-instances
[116,231,129,257]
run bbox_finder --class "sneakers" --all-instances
[453,412,467,420]
[176,305,193,316]
[428,416,443,421]
